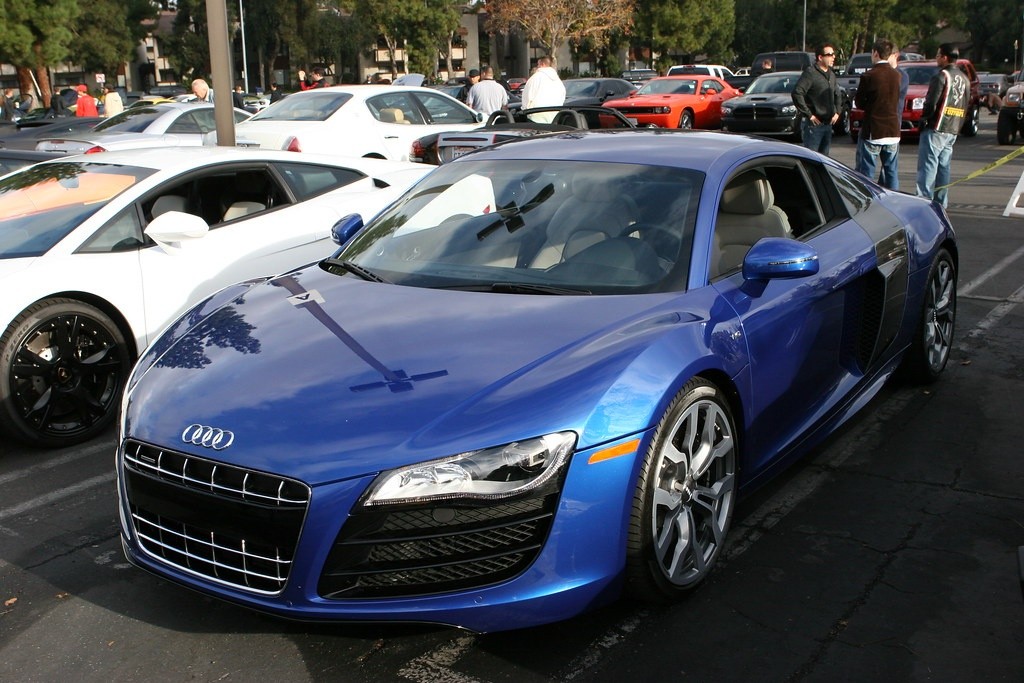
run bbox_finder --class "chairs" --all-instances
[151,179,194,219]
[222,171,277,221]
[528,168,640,270]
[380,107,411,124]
[669,169,793,292]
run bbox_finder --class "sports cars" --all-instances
[0,116,114,135]
[720,72,851,141]
[407,104,659,174]
[113,130,958,634]
[0,144,503,434]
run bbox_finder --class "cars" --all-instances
[0,99,252,177]
[377,72,521,117]
[505,77,637,130]
[599,74,744,133]
[202,83,490,162]
[977,71,1019,95]
[623,69,658,88]
[67,95,270,113]
[733,66,750,74]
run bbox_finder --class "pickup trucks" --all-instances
[836,53,927,92]
[724,50,818,92]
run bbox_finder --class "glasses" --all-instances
[821,53,834,56]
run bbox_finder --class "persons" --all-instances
[362,75,383,84]
[980,93,1002,115]
[455,56,566,124]
[233,85,244,110]
[50,83,124,118]
[854,38,909,192]
[191,79,214,104]
[760,59,775,74]
[915,43,971,209]
[270,83,282,104]
[298,67,330,91]
[791,43,842,156]
[656,69,667,77]
[3,88,20,121]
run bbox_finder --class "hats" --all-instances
[73,84,87,91]
[100,83,114,89]
[467,69,479,77]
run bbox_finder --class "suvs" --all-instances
[849,60,982,140]
[667,65,736,87]
[997,66,1024,144]
